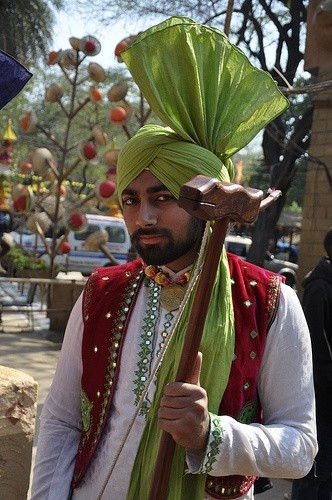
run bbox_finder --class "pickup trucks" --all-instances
[9,215,133,278]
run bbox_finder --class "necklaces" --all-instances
[141,259,194,312]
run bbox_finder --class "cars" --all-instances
[222,236,300,289]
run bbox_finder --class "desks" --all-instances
[0,276,88,332]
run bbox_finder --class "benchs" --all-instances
[0,309,48,313]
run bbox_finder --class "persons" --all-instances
[28,123,319,500]
[291,229,332,500]
